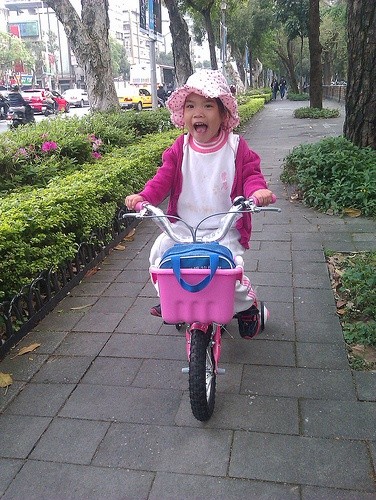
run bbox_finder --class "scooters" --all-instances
[157,90,172,108]
[6,100,36,131]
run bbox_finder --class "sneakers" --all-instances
[236,300,261,341]
[150,303,162,318]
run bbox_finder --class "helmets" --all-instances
[158,83,164,88]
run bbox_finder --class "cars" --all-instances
[0,85,70,120]
[119,88,152,111]
[61,88,89,108]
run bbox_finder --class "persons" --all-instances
[44,88,57,113]
[0,86,32,122]
[271,77,286,100]
[125,70,273,339]
[157,86,169,104]
[303,82,307,92]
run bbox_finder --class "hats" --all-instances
[164,69,241,132]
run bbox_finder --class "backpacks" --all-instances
[159,242,236,292]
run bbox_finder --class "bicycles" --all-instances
[121,193,283,422]
